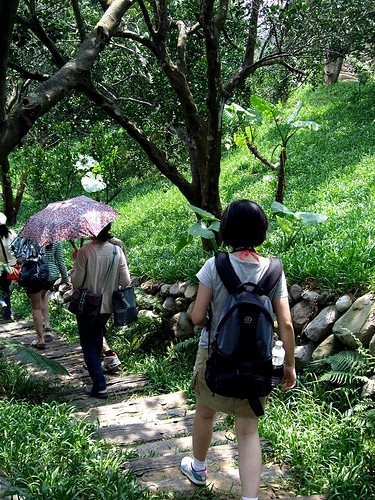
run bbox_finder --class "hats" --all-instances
[0,212,7,225]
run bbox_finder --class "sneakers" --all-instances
[180,456,207,485]
[101,352,122,371]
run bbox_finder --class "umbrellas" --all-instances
[17,195,120,250]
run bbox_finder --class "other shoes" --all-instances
[83,386,108,399]
[3,314,14,321]
[43,328,53,342]
[32,339,47,347]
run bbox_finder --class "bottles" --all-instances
[272,341,285,386]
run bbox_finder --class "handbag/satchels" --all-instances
[68,288,102,323]
[112,286,139,326]
[6,266,21,280]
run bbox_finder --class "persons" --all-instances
[180,199,296,500]
[0,212,132,398]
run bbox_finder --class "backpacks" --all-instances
[206,250,286,418]
[18,256,49,286]
